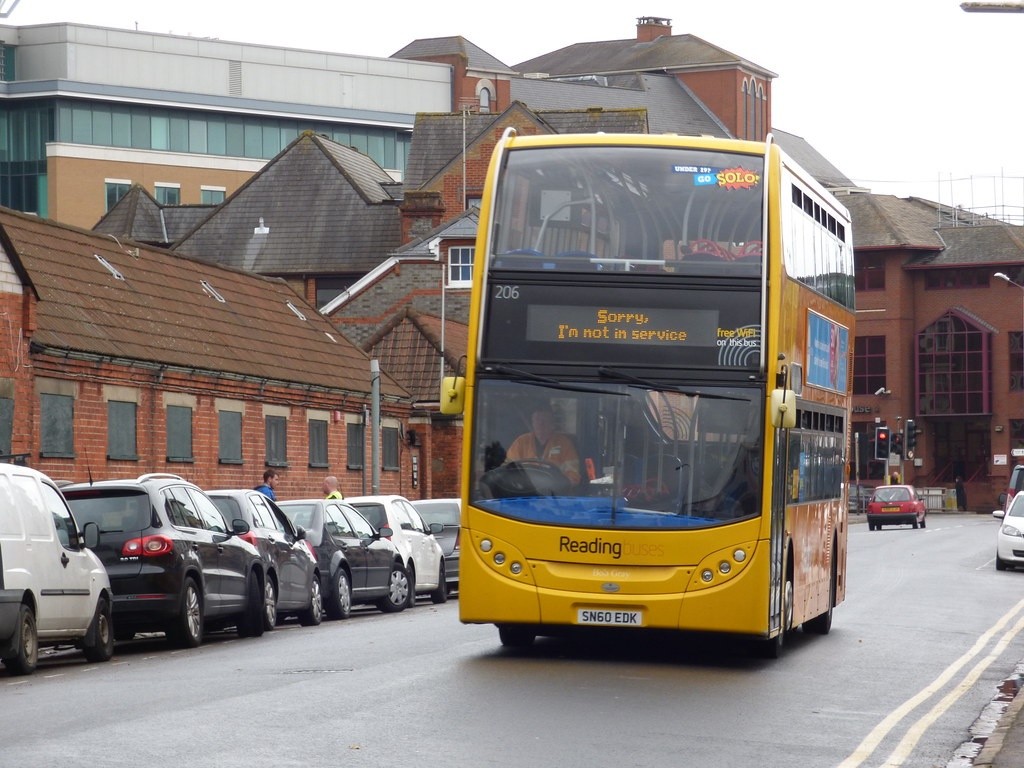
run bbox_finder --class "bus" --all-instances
[440,127,856,660]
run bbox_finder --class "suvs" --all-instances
[50,473,266,648]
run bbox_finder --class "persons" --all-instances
[501,408,582,492]
[891,471,899,484]
[253,470,279,502]
[955,476,967,512]
[322,476,343,500]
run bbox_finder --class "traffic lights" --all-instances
[875,427,890,460]
[895,435,902,454]
[905,420,917,460]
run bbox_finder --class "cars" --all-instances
[202,489,322,631]
[275,499,411,619]
[993,491,1024,571]
[345,494,447,608]
[849,484,875,511]
[867,485,926,530]
[409,498,461,594]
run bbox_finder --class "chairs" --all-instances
[500,250,763,278]
[480,453,762,521]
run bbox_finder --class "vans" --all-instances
[0,463,113,675]
[998,465,1024,515]
